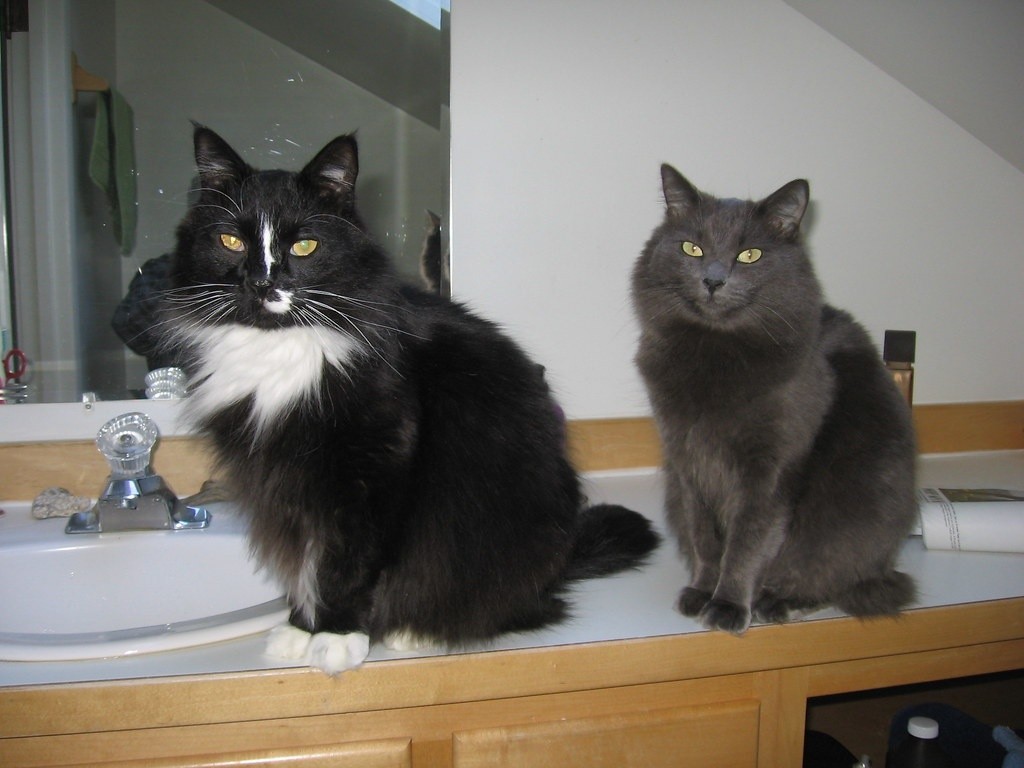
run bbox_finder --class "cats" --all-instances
[622,163,923,635]
[122,119,663,676]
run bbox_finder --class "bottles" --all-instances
[886,716,955,768]
[882,330,916,408]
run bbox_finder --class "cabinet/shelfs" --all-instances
[0,669,780,768]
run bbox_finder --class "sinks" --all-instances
[1,527,296,659]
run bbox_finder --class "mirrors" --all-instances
[0,0,451,405]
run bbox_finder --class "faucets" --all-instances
[66,412,212,534]
[143,368,188,400]
